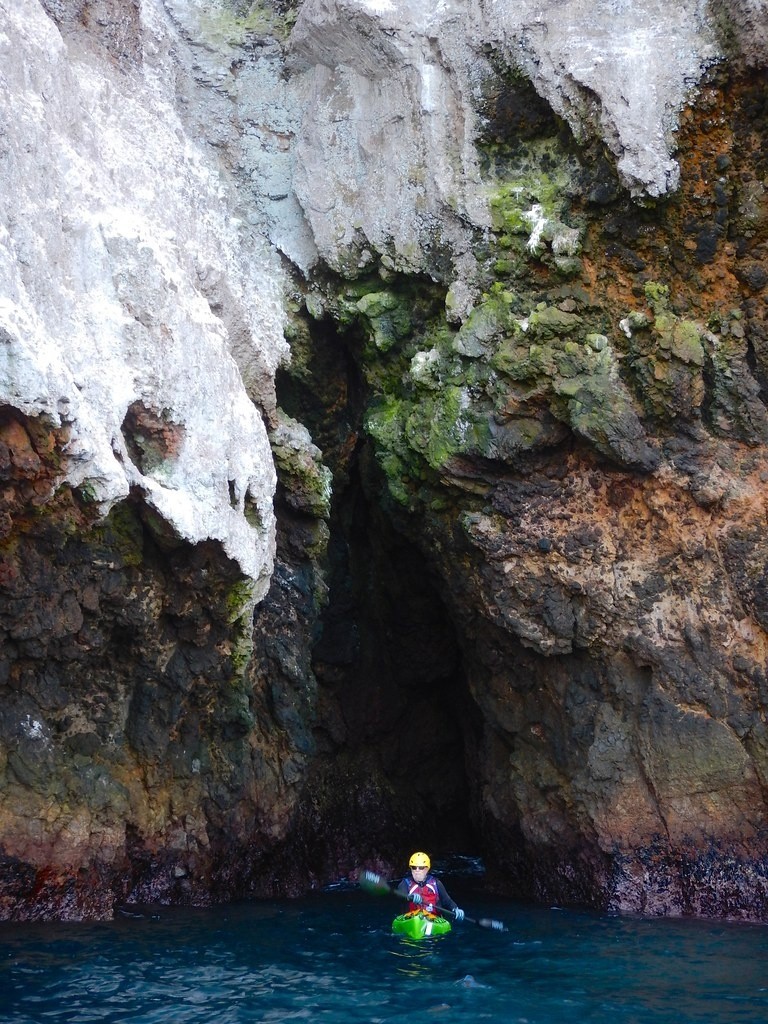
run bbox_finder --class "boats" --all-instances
[392,912,452,941]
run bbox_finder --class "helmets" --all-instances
[409,852,431,868]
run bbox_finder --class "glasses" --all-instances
[411,866,425,870]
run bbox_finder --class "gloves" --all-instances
[407,893,422,904]
[452,907,465,921]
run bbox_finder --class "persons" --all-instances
[398,853,464,921]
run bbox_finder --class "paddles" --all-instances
[356,869,509,934]
